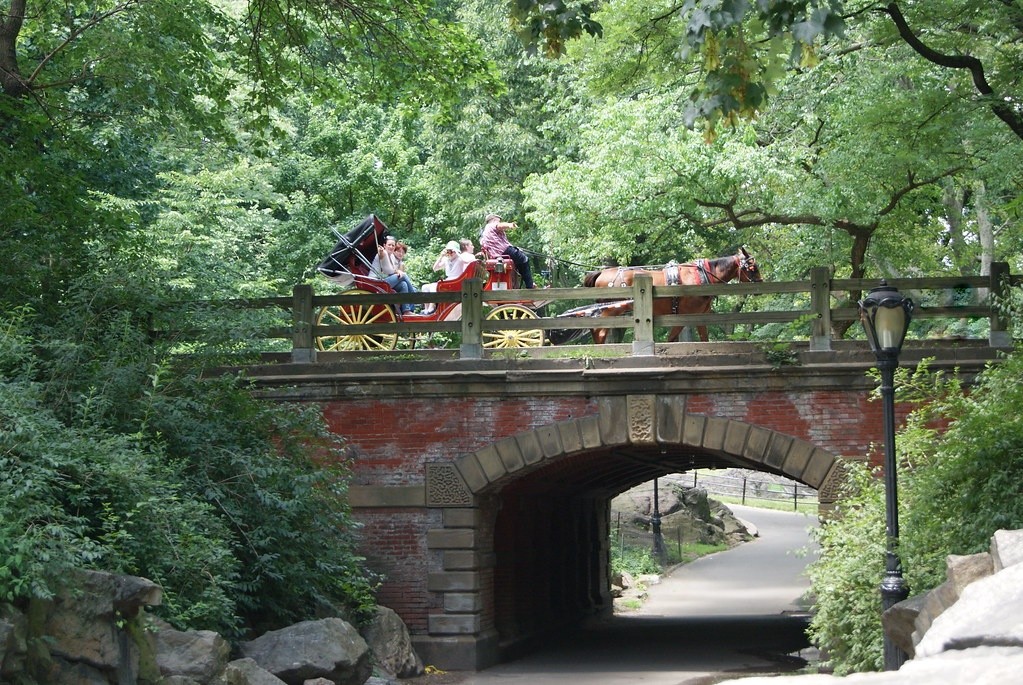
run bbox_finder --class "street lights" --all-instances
[858,278,915,674]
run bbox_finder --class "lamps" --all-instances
[659,442,671,456]
[688,453,695,464]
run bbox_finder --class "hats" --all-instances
[486,214,503,222]
[445,240,460,252]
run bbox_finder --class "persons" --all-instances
[479,212,537,289]
[420,237,476,314]
[368,235,419,316]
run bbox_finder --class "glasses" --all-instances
[384,236,396,241]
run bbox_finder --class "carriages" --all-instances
[315,214,763,352]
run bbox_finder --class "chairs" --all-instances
[481,243,515,269]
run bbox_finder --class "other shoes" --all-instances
[421,307,436,315]
[404,311,416,314]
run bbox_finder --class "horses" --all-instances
[582,247,765,344]
